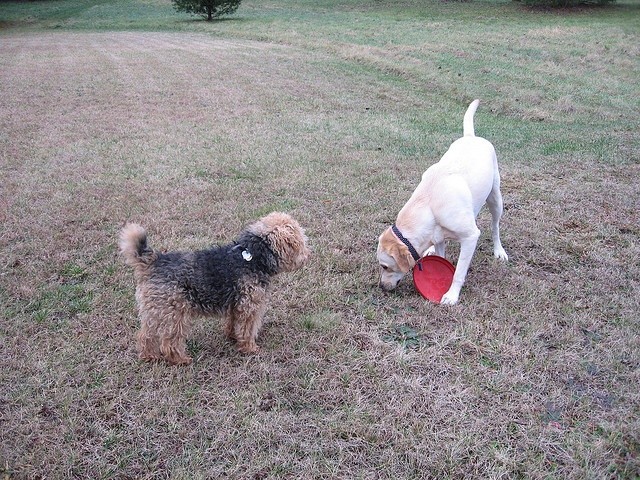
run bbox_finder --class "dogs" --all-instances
[377,97,511,306]
[118,210,311,365]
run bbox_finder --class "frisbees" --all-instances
[414,256,455,305]
